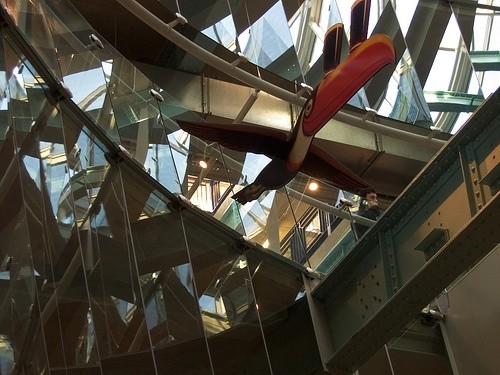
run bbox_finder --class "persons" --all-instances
[352,188,379,237]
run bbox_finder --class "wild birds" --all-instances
[229,33,395,205]
